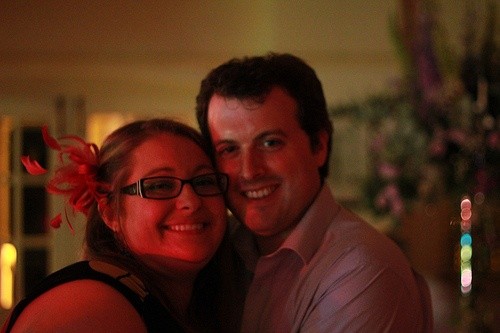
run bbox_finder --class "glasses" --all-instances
[120,172,230,200]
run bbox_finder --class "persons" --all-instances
[1,118,228,333]
[196,54,431,332]
